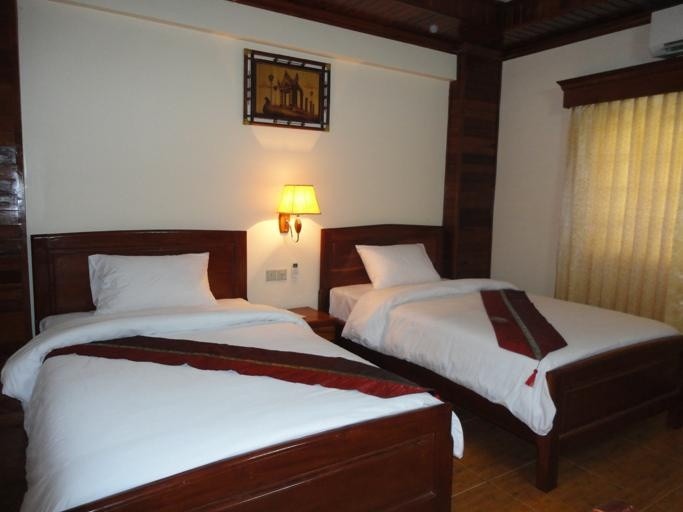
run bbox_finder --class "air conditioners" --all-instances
[649,4,683,58]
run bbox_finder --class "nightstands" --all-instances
[283,307,337,344]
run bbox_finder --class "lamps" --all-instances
[274,184,322,242]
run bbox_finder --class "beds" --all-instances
[317,224,682,491]
[31,228,453,511]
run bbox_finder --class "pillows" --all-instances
[86,252,215,316]
[354,242,443,289]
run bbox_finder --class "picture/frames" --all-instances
[241,48,332,133]
